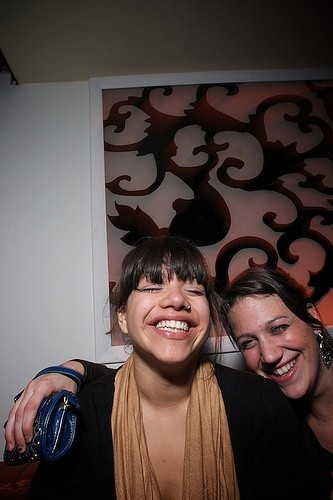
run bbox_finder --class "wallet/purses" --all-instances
[2,365,84,467]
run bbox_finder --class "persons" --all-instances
[5,263,333,500]
[31,233,329,500]
